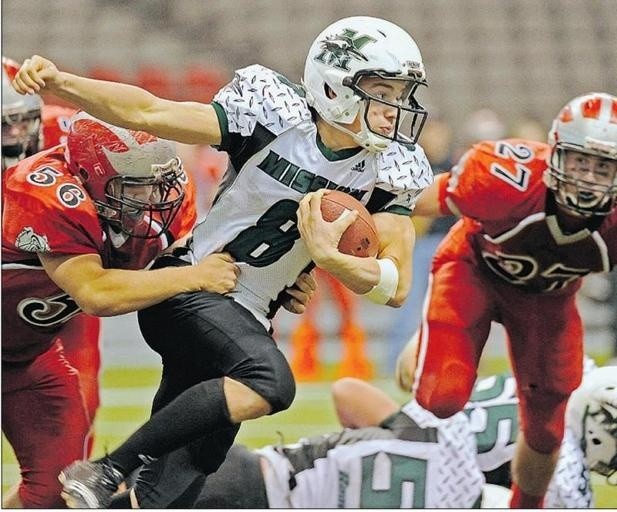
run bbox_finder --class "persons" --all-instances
[464,355,617,510]
[396,92,617,510]
[194,378,513,512]
[1,108,318,510]
[2,55,101,422]
[11,16,434,510]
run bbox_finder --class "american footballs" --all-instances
[309,190,380,259]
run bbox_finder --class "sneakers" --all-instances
[58,459,125,509]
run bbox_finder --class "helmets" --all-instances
[303,16,426,124]
[64,108,184,220]
[542,92,617,190]
[1,57,44,152]
